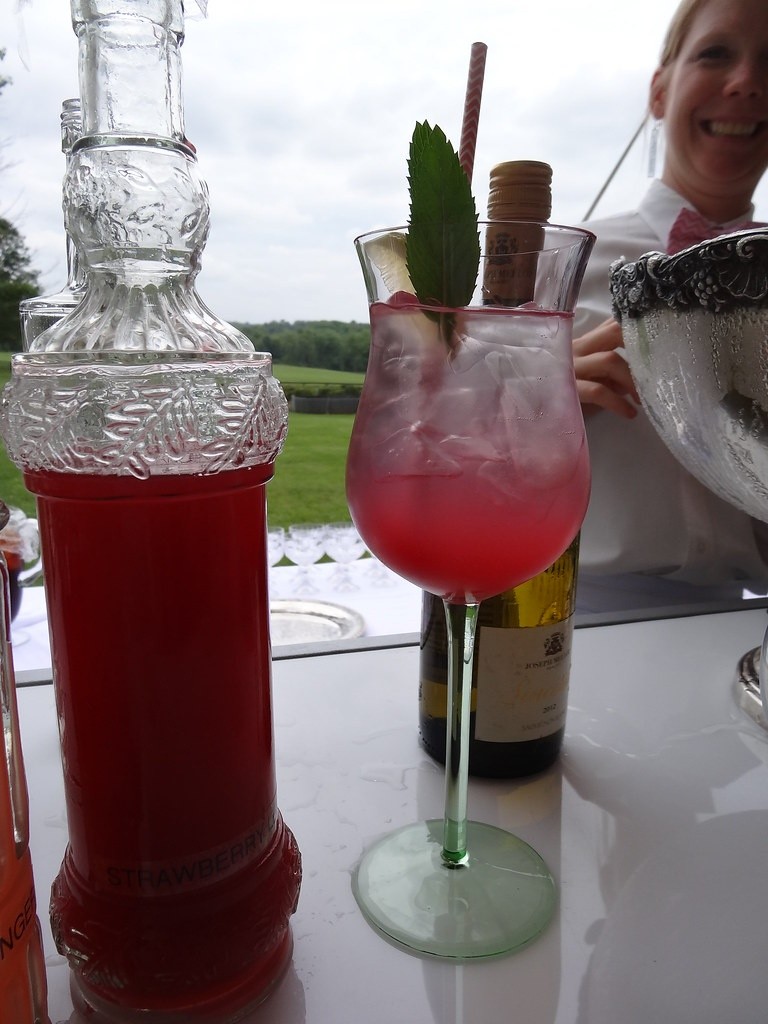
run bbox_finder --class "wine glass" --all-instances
[346,221,597,961]
[609,224,765,731]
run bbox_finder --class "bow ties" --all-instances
[666,207,768,255]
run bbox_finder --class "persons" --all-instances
[540,4,767,581]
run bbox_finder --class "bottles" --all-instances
[1,513,50,1019]
[16,98,92,358]
[420,161,580,780]
[6,0,301,1023]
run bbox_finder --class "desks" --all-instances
[12,591,768,1020]
